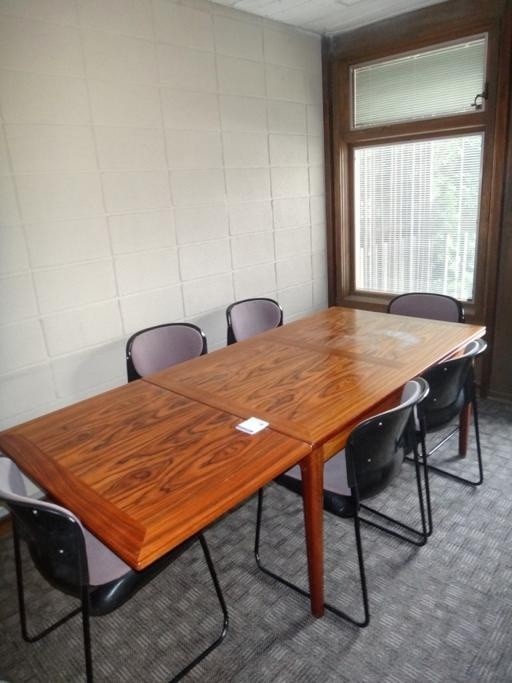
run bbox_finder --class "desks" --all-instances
[2,307,488,618]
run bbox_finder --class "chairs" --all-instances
[125,293,284,383]
[360,293,488,537]
[254,375,428,627]
[2,457,229,681]
